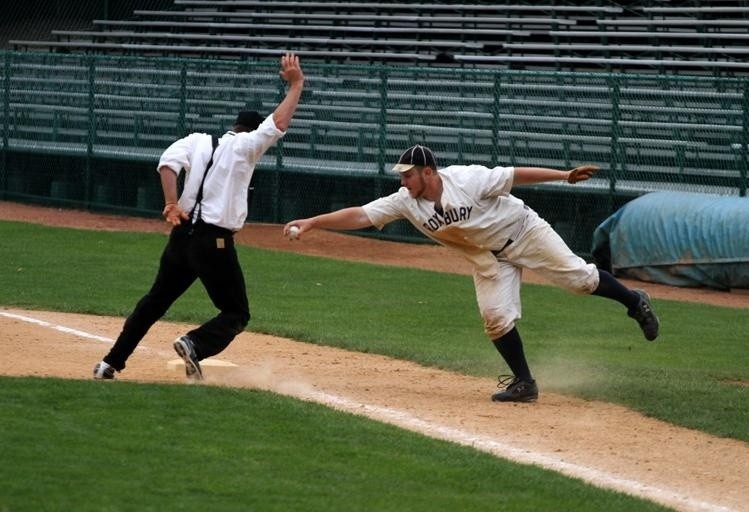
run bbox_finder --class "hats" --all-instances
[235,111,266,129]
[391,144,436,173]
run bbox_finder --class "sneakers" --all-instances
[173,334,203,381]
[627,288,661,342]
[93,360,118,381]
[492,378,539,402]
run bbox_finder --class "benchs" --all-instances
[1,0,747,187]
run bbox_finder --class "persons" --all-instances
[282,145,662,403]
[92,51,305,381]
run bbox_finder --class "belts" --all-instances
[490,239,513,256]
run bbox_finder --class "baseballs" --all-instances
[287,226,299,240]
